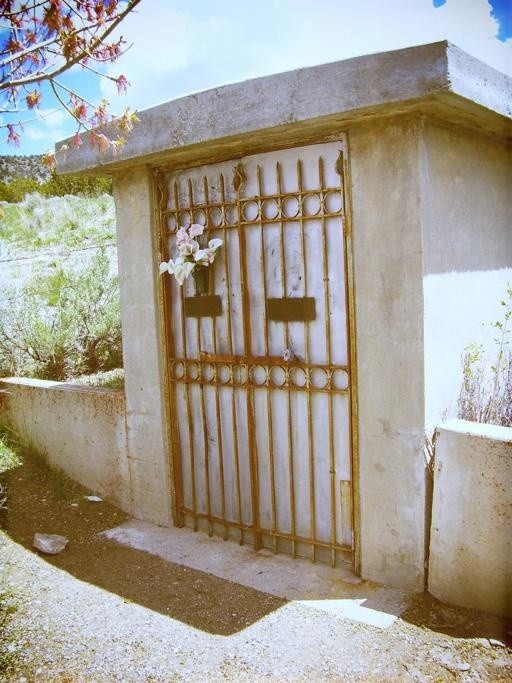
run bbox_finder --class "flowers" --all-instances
[160,222,222,297]
